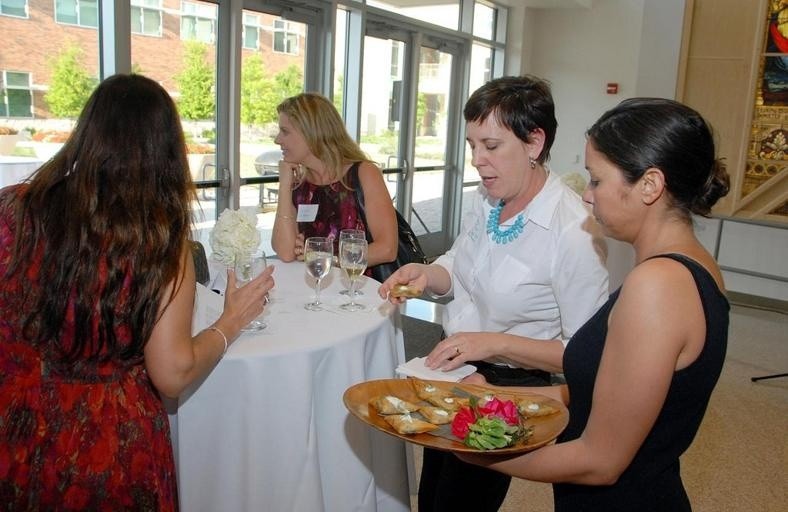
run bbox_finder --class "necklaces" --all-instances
[484,196,527,246]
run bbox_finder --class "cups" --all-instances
[339,229,364,297]
[340,238,365,312]
[232,248,267,333]
[304,237,334,312]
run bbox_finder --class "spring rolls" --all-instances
[412,376,455,400]
[391,284,422,299]
[369,395,421,414]
[384,414,440,435]
[428,397,471,411]
[515,396,560,418]
[418,406,457,425]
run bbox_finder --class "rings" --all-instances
[261,295,270,308]
[453,345,461,356]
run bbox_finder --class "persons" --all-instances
[378,76,610,511]
[409,98,729,512]
[0,73,275,511]
[269,93,399,286]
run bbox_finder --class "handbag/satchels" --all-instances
[351,158,429,286]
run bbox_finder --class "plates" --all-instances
[343,378,571,455]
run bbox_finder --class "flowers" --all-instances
[208,206,260,265]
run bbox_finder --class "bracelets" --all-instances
[273,210,299,226]
[199,324,230,357]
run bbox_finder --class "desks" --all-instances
[174,252,400,512]
